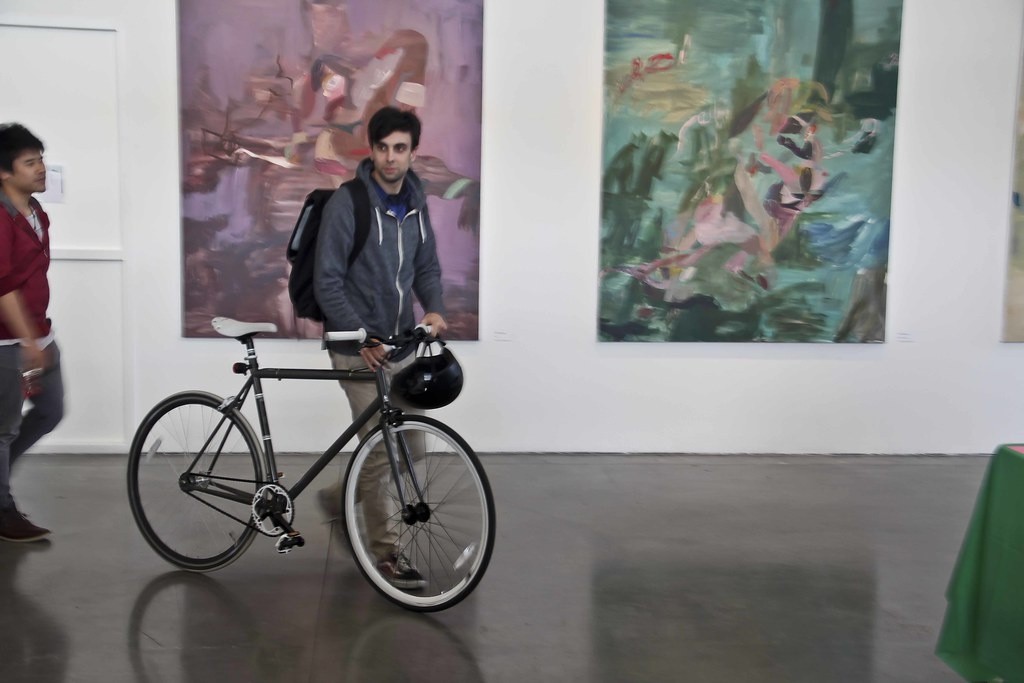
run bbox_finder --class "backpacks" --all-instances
[285,180,371,323]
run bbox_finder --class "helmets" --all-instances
[391,351,463,410]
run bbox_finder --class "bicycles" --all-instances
[126,317,499,613]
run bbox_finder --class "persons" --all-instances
[317,105,449,592]
[1,121,68,542]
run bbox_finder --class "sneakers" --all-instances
[316,489,355,555]
[0,506,54,543]
[376,550,429,590]
